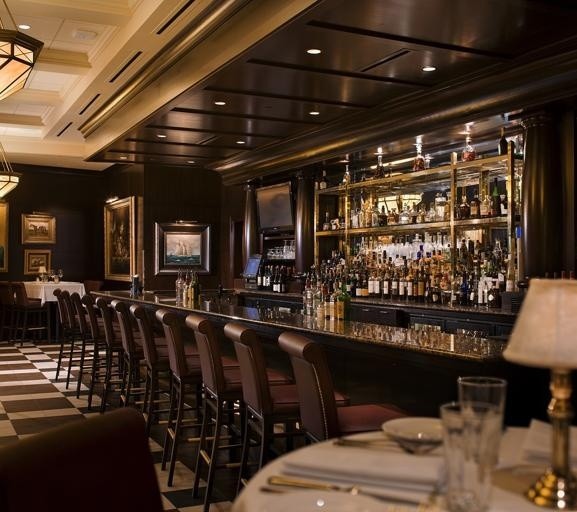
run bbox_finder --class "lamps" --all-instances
[0,141,22,199]
[505,278,577,511]
[38,265,47,282]
[0,0,44,103]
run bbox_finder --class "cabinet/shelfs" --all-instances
[310,139,524,302]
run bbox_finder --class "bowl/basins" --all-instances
[383,416,443,454]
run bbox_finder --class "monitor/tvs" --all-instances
[243,254,264,279]
[255,181,296,235]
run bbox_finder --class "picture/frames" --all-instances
[0,201,9,271]
[24,249,50,273]
[104,195,136,280]
[154,221,211,274]
[22,212,56,245]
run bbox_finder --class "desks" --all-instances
[241,411,576,512]
[19,280,85,340]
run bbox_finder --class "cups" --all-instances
[457,375,507,467]
[437,399,502,512]
[138,285,144,293]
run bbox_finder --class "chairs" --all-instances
[11,282,44,347]
[1,281,22,348]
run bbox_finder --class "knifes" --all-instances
[268,474,420,508]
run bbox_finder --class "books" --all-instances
[520,420,577,460]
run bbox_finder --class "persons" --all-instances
[111,222,129,258]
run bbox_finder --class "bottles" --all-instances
[175,266,201,303]
[254,307,294,327]
[303,231,510,320]
[128,292,144,300]
[173,298,200,310]
[132,274,138,293]
[318,125,508,230]
[298,316,491,358]
[257,261,290,294]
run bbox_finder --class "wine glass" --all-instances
[49,268,64,282]
[266,238,296,260]
